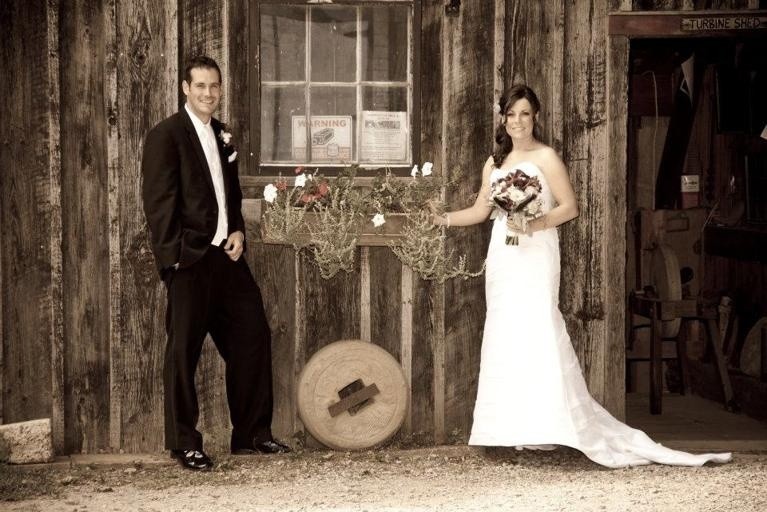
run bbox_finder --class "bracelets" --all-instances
[446,212,450,229]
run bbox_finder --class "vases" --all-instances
[258,208,445,250]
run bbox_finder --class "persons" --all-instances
[140,55,292,471]
[427,83,734,469]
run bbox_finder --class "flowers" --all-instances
[486,169,546,247]
[262,161,486,282]
[219,126,234,147]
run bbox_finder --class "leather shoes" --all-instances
[231,428,291,455]
[173,448,213,472]
